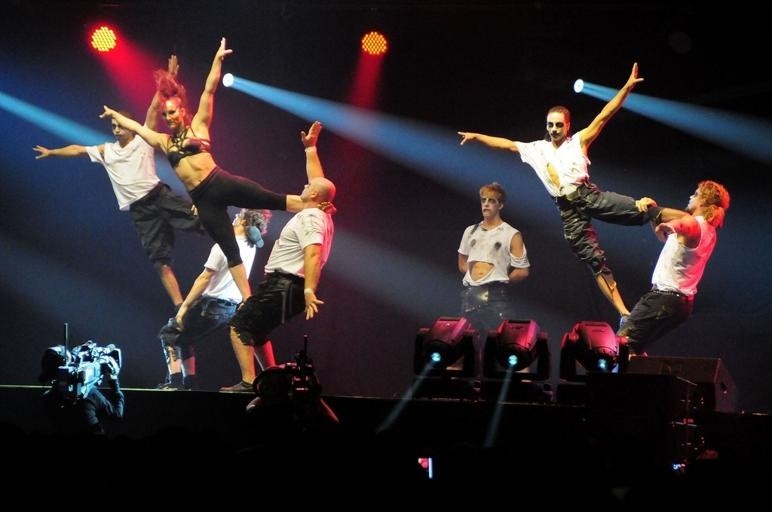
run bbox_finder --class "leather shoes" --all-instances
[220,381,254,392]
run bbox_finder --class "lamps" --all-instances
[414,318,632,401]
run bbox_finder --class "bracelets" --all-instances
[302,287,317,295]
[304,145,318,154]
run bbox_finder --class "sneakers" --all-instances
[158,382,184,391]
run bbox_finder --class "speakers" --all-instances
[586,372,698,413]
[628,354,741,413]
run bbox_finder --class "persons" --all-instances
[154,201,271,391]
[608,176,731,371]
[451,181,529,390]
[37,347,129,512]
[454,62,727,338]
[32,52,266,313]
[217,119,339,394]
[95,32,339,310]
